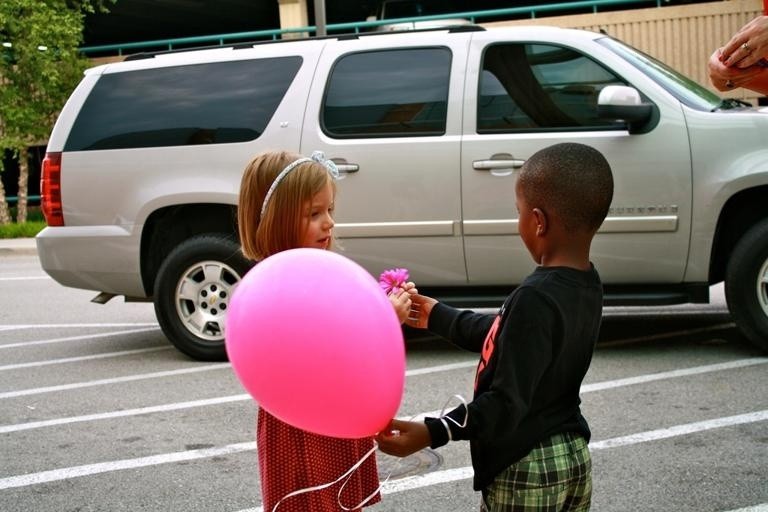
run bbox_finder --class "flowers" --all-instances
[379,267,409,297]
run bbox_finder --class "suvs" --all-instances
[35,25,767,362]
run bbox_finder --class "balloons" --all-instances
[225,248,406,440]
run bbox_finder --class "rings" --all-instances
[725,79,736,89]
[741,41,754,54]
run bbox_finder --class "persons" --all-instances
[236,148,383,512]
[375,143,615,511]
[707,1,768,99]
